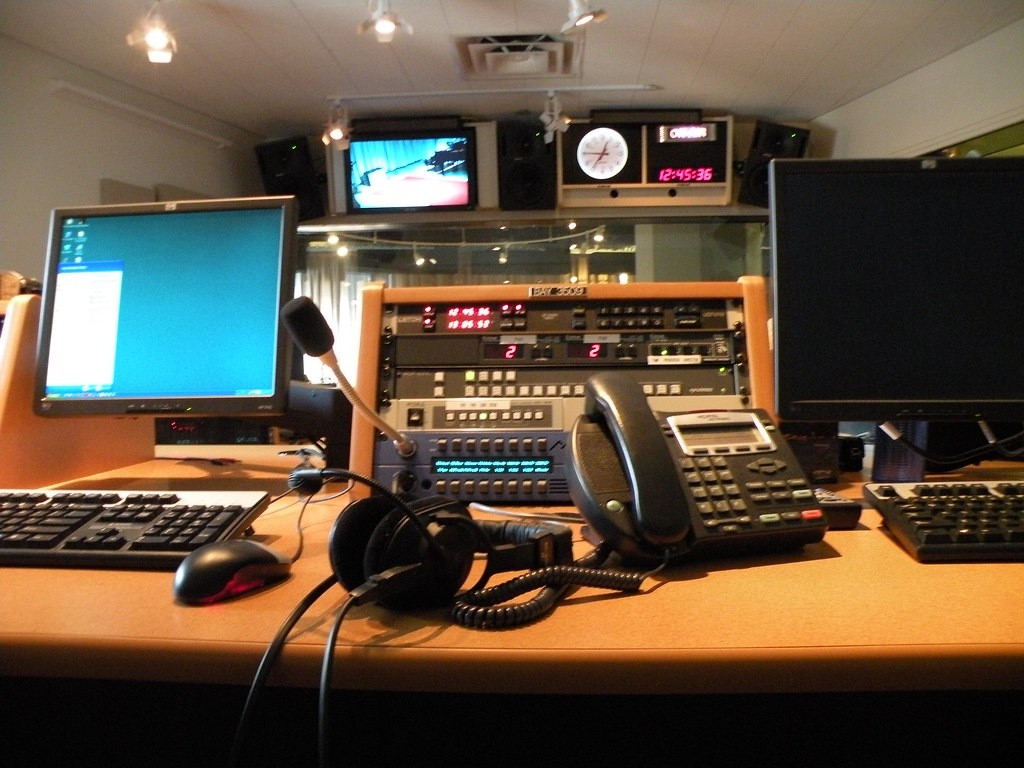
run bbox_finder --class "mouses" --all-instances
[174,539,291,607]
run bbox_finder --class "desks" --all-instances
[0,461,1023,693]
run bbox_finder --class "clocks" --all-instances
[576,127,629,181]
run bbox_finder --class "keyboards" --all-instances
[862,480,1024,564]
[0,489,274,576]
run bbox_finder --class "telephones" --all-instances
[564,371,831,569]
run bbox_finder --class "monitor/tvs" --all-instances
[33,195,298,417]
[344,125,480,215]
[770,157,1024,485]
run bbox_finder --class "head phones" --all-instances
[330,494,574,614]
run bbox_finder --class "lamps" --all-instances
[324,99,352,150]
[539,91,571,145]
[358,1,412,42]
[559,0,607,36]
[124,0,179,64]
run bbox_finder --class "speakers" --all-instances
[255,135,326,222]
[497,121,557,210]
[737,120,811,209]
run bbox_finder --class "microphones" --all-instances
[280,296,416,459]
[289,467,449,571]
